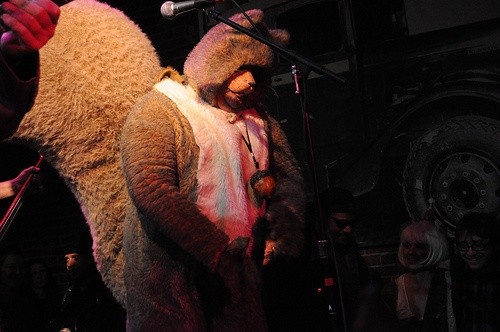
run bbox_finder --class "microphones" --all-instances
[160,0,234,18]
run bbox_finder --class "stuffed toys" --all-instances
[120,8,306,332]
[0,0,210,332]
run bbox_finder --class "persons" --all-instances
[273,184,500,332]
[0,0,126,332]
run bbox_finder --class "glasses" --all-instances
[458,239,494,252]
[330,216,359,228]
